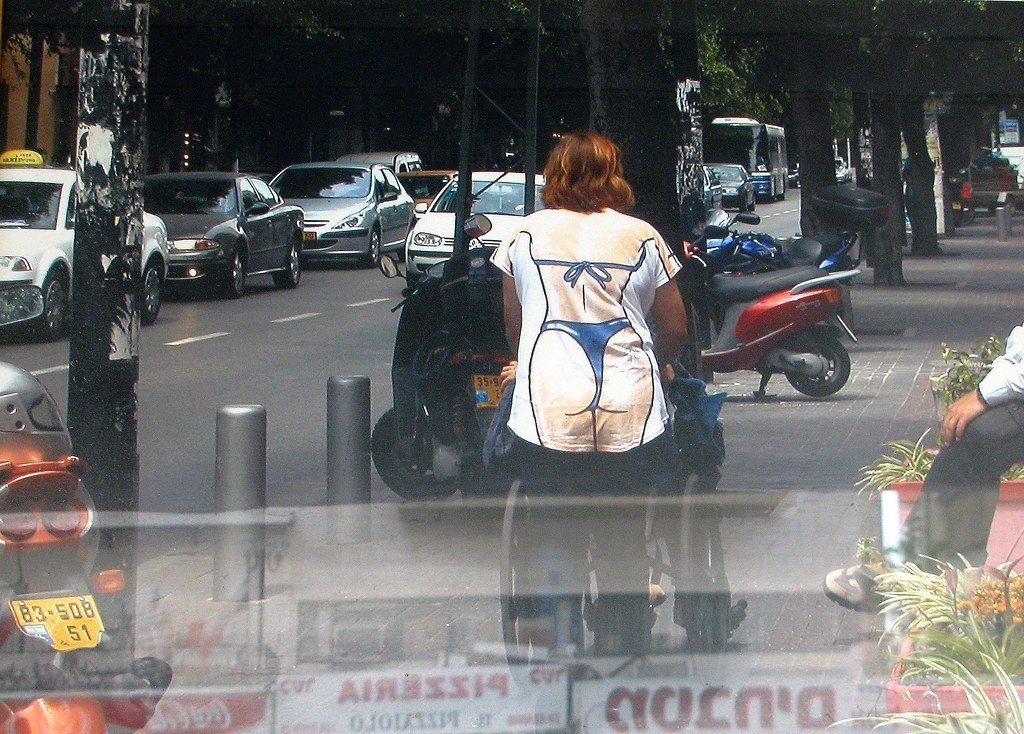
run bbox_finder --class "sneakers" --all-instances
[823,562,886,612]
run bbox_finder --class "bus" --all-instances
[707,115,790,200]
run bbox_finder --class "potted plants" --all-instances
[851,330,1024,734]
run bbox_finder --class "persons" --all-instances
[490,130,690,661]
[823,319,1024,612]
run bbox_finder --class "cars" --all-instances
[409,171,560,272]
[835,156,852,183]
[702,160,750,207]
[0,147,169,335]
[269,149,455,264]
[145,169,305,293]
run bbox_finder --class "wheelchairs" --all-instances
[493,371,738,673]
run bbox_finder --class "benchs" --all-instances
[970,166,1024,214]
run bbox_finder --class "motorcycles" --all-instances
[375,256,512,504]
[681,180,889,268]
[0,361,171,732]
[678,250,859,400]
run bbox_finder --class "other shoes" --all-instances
[535,695,581,734]
[673,599,686,629]
[593,583,667,628]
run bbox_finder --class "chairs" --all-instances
[0,194,33,221]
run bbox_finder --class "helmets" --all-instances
[0,361,73,465]
